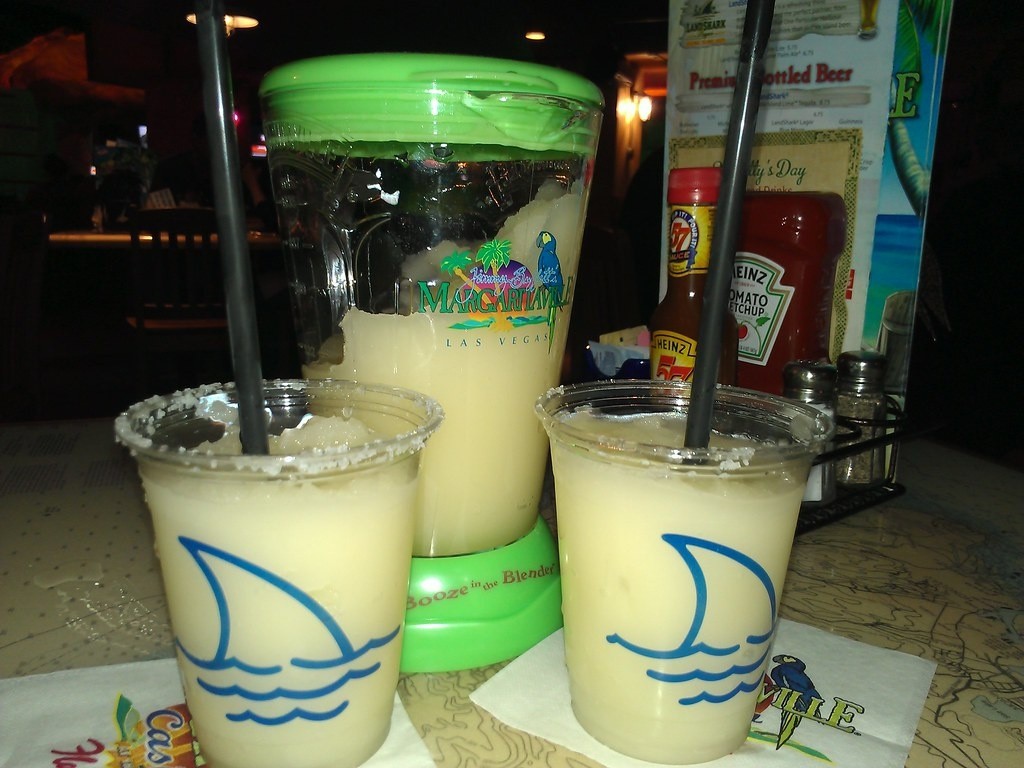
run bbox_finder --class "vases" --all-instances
[95,171,147,231]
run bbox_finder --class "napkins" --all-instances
[469,617,937,768]
[0,658,438,768]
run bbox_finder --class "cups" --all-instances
[114,377,443,767]
[533,378,836,765]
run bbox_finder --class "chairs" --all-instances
[0,200,48,423]
[123,207,230,404]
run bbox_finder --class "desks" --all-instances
[0,416,1024,768]
[49,230,282,251]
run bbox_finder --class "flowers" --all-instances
[90,146,151,178]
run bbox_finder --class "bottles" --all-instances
[838,352,886,488]
[644,165,740,402]
[728,190,846,396]
[781,362,838,506]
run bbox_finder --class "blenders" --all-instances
[257,52,603,673]
